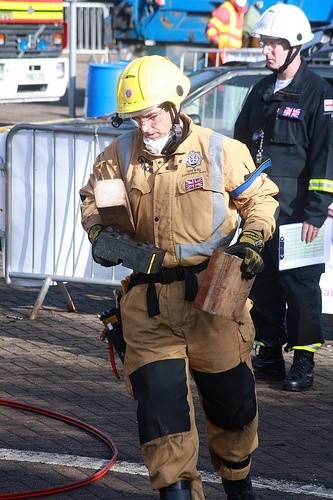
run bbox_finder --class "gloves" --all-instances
[224,231,264,280]
[87,224,128,268]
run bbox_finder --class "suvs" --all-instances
[0,56,333,139]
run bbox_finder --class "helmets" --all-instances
[249,3,314,46]
[115,55,191,118]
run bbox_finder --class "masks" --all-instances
[235,0,247,6]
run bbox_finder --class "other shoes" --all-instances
[157,480,190,500]
[221,470,255,500]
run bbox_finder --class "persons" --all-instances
[232,3,333,390]
[204,0,248,119]
[79,55,281,500]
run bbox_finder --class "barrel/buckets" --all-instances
[85,63,125,119]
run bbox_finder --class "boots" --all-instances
[281,349,315,391]
[250,346,284,371]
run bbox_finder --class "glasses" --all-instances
[129,107,164,128]
[256,39,287,48]
